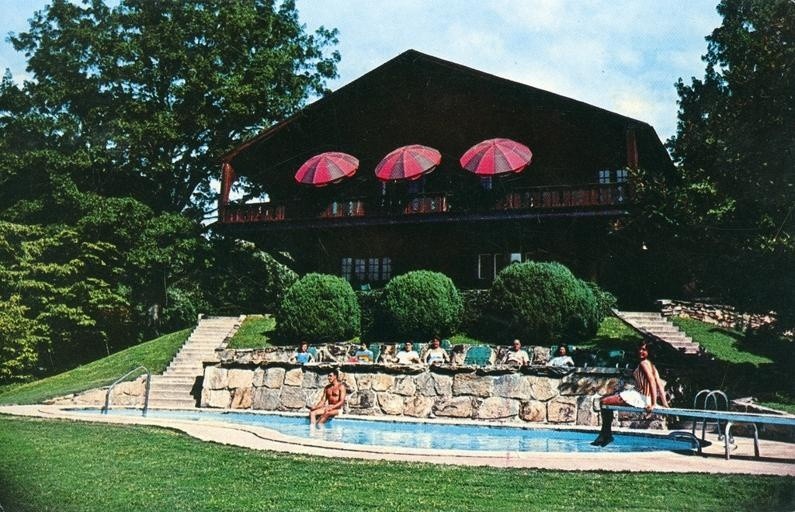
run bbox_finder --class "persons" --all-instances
[545,345,576,366]
[500,338,530,368]
[422,334,452,363]
[384,339,420,364]
[308,368,346,427]
[290,340,316,363]
[591,337,671,447]
[353,340,374,364]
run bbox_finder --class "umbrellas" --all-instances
[373,144,443,186]
[291,151,361,188]
[459,138,537,191]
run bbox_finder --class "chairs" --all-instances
[368,339,625,368]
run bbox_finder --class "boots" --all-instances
[591,409,614,446]
